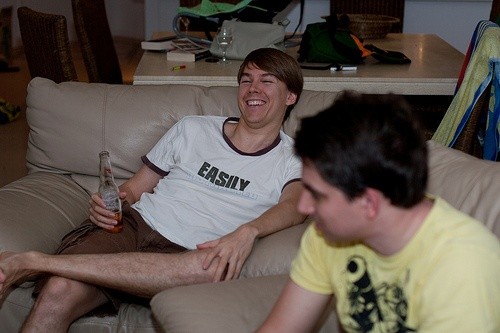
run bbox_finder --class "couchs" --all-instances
[0,77,339,333]
[150,140,500,333]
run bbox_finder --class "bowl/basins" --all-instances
[321,14,399,41]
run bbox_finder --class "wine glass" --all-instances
[216,25,233,65]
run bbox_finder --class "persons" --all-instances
[253,88,500,332]
[0,47,309,333]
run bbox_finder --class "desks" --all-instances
[133,33,466,94]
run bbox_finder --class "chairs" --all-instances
[17,7,79,83]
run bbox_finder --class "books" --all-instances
[141,35,212,61]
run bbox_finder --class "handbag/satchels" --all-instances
[210,18,285,61]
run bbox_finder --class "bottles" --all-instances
[98,150,122,233]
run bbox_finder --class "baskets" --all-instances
[320,13,400,40]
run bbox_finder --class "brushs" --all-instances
[350,33,380,64]
